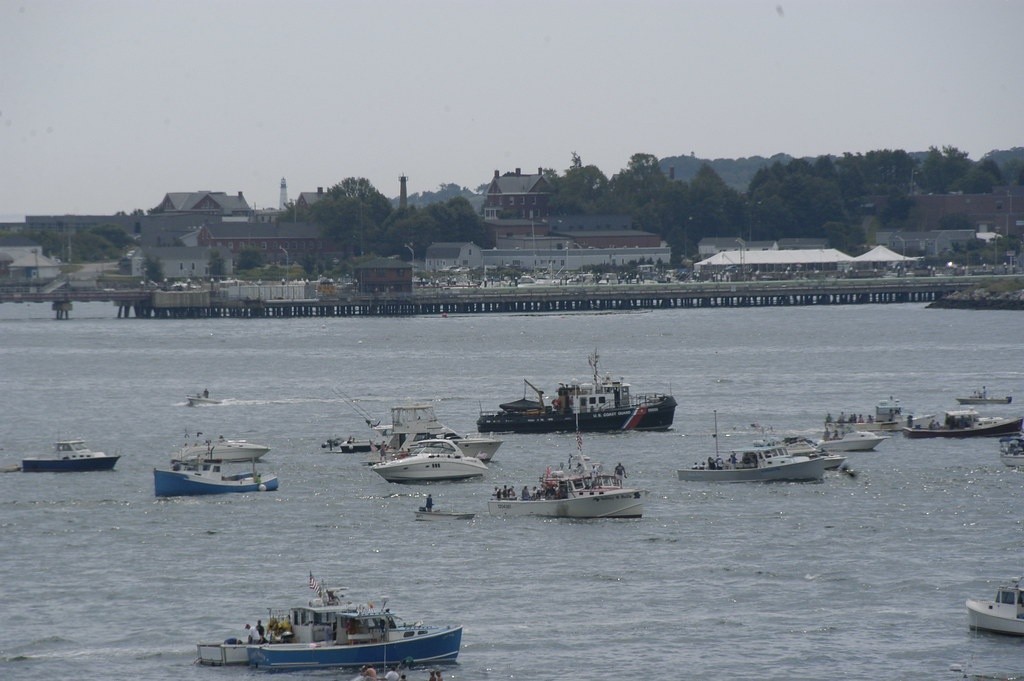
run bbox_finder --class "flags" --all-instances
[309,574,321,595]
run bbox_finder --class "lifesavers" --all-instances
[552,400,559,407]
[596,464,603,472]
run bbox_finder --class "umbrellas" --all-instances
[851,245,916,270]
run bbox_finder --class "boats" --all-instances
[167,440,273,466]
[188,395,221,408]
[485,464,644,519]
[999,439,1024,468]
[475,380,680,433]
[772,437,846,471]
[367,405,502,462]
[965,573,1024,638]
[675,444,826,483]
[341,438,371,454]
[153,466,282,495]
[955,385,1012,405]
[194,600,463,669]
[900,410,1023,439]
[22,438,122,472]
[370,439,489,485]
[814,421,891,452]
[845,414,936,433]
[412,508,474,520]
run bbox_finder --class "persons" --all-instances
[905,411,976,431]
[323,620,334,644]
[245,620,270,643]
[203,388,210,398]
[379,439,411,464]
[361,664,442,681]
[590,464,598,483]
[426,493,433,513]
[823,412,873,440]
[888,407,901,420]
[615,463,627,489]
[690,451,737,471]
[492,484,560,501]
[973,385,987,399]
